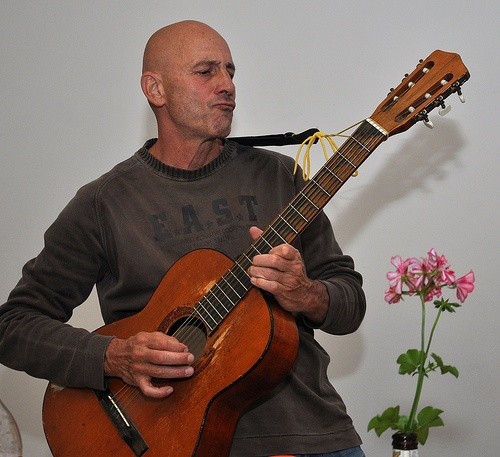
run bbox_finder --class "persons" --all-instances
[0,20,368,457]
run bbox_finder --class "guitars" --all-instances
[40,48,470,457]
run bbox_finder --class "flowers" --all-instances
[367,248,475,445]
